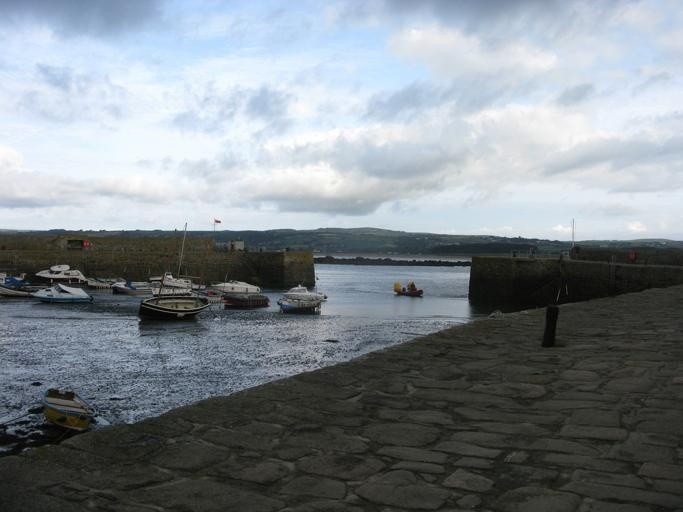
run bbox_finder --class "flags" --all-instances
[214,218,221,224]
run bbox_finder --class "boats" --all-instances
[0,265,269,318]
[278,284,326,312]
[394,281,423,296]
[43,388,96,431]
